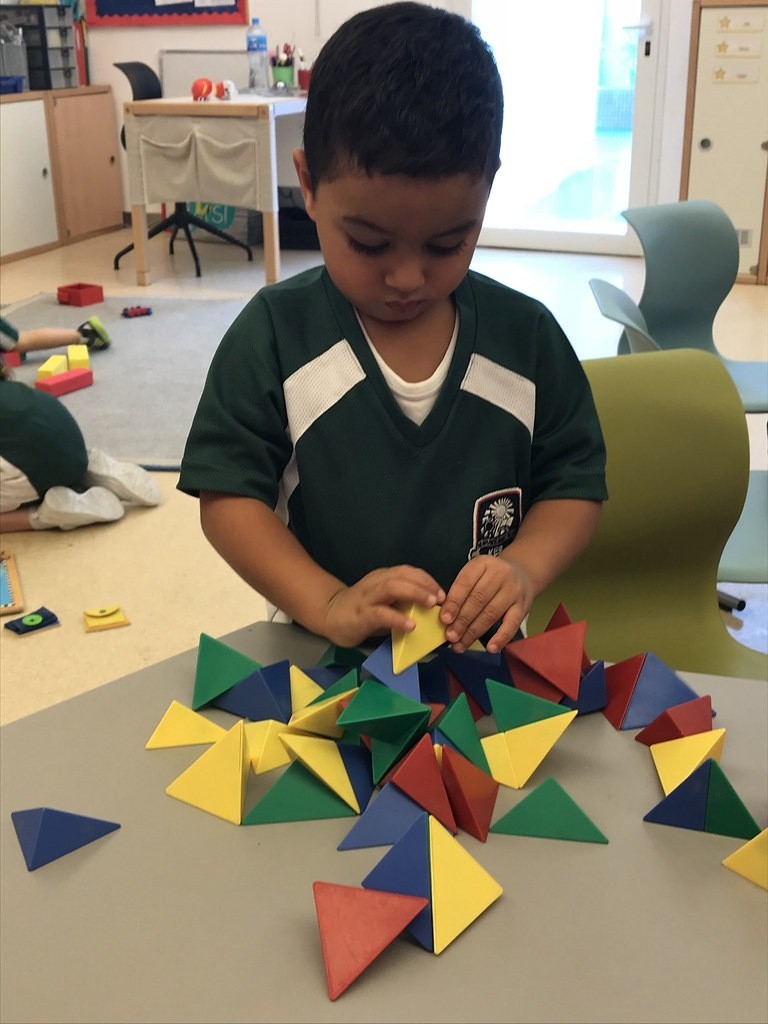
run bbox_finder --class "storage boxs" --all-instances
[0,44,30,93]
[58,283,103,306]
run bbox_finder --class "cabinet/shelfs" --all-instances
[111,62,253,276]
[0,85,123,266]
[0,4,77,89]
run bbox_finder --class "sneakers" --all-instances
[78,316,112,350]
[83,448,161,508]
[30,485,125,529]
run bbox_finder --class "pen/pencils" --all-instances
[270,43,296,66]
[298,48,306,69]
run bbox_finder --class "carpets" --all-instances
[0,292,254,475]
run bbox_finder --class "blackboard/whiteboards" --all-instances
[86,0,249,26]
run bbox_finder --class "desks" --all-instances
[123,91,306,286]
[0,619,768,1024]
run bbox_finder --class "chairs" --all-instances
[588,276,766,611]
[523,350,766,680]
[616,199,767,414]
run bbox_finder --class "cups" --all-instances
[272,66,293,89]
[298,70,311,90]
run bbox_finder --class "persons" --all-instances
[0,381,162,532]
[177,2,610,660]
[0,316,110,360]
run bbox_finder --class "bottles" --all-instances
[246,18,270,90]
